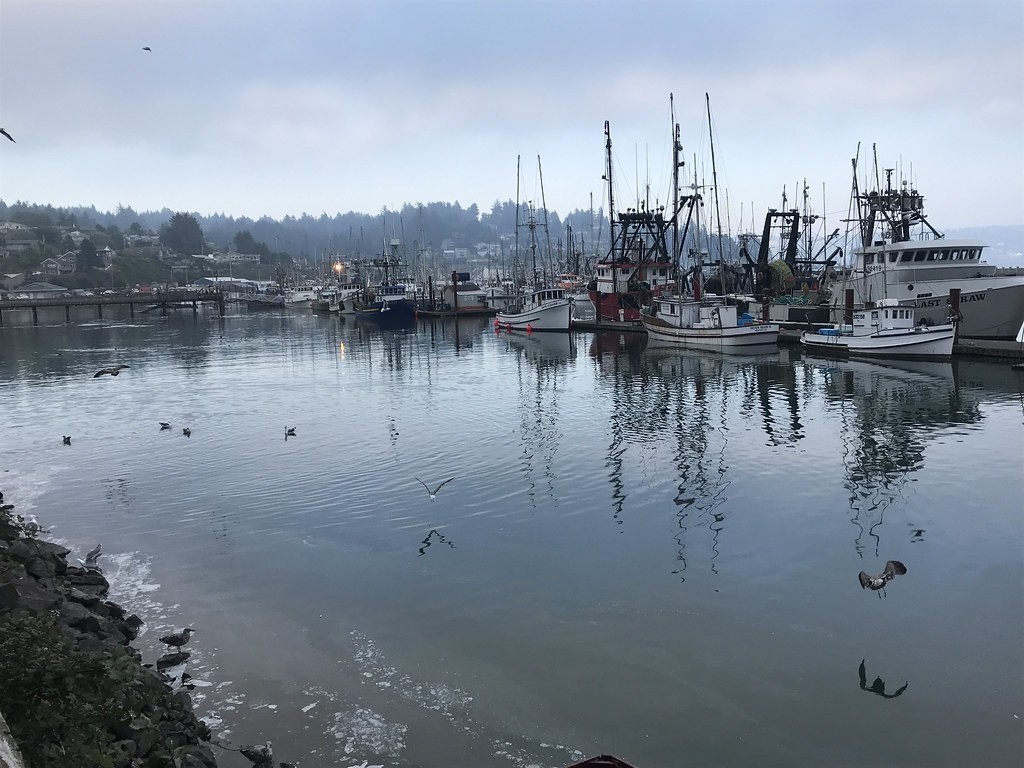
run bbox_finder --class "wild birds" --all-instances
[159,421,172,428]
[94,365,130,378]
[159,628,196,653]
[25,514,38,537]
[284,426,296,433]
[0,128,16,143]
[240,740,273,768]
[414,476,455,502]
[183,428,191,437]
[858,559,907,600]
[143,47,151,52]
[63,436,71,442]
[75,543,104,574]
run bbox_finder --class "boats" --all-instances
[800,299,962,359]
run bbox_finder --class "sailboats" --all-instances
[289,89,1024,360]
